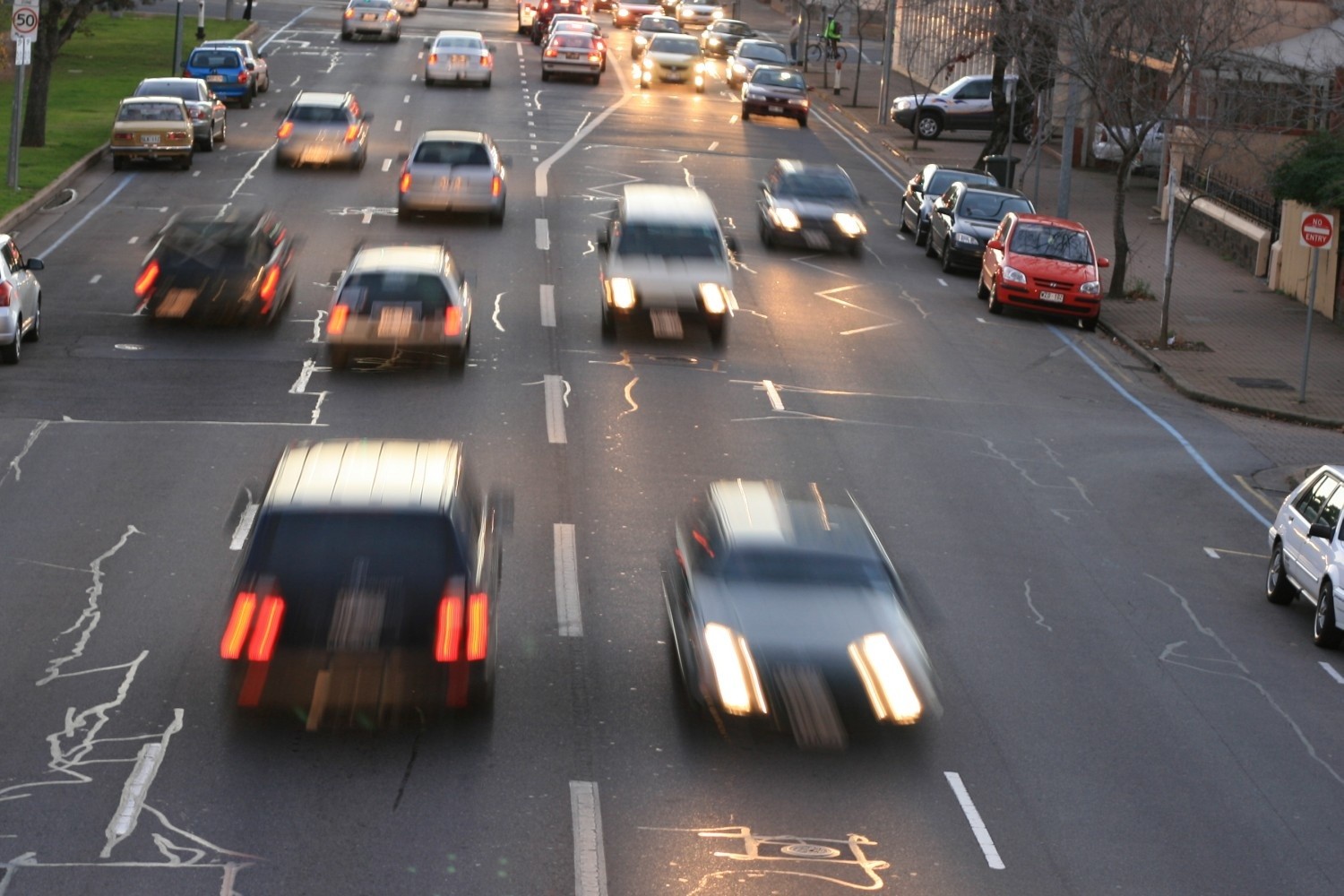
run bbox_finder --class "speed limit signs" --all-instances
[11,7,38,35]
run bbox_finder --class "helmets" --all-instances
[827,14,833,20]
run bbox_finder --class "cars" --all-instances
[318,245,476,369]
[0,233,46,357]
[108,39,271,172]
[1266,465,1344,649]
[395,131,516,227]
[892,76,1047,147]
[138,204,297,328]
[340,0,791,95]
[901,165,1039,272]
[759,159,870,253]
[979,211,1111,333]
[740,65,813,131]
[1092,122,1165,178]
[595,183,737,354]
[660,480,940,740]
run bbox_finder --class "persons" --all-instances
[788,16,799,65]
[823,14,841,61]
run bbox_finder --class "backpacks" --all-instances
[832,22,841,35]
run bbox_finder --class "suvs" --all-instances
[273,89,372,173]
[213,449,527,739]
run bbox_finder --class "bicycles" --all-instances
[807,34,847,63]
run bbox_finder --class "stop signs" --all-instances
[1302,213,1332,247]
